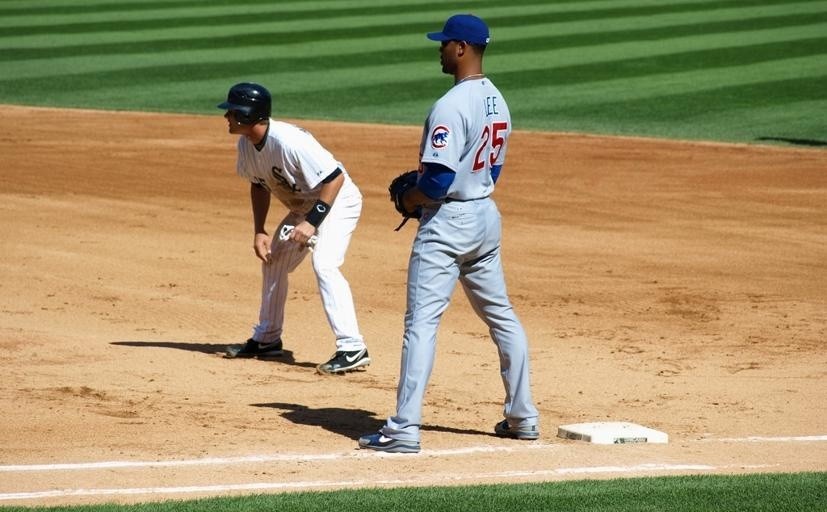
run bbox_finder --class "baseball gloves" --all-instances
[389,170,421,220]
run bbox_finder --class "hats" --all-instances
[427,12,488,47]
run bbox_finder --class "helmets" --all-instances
[216,82,273,126]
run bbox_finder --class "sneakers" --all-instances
[494,419,540,440]
[318,346,370,374]
[358,434,419,451]
[224,338,283,357]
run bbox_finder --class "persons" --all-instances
[359,14,544,453]
[216,82,370,377]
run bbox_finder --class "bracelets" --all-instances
[303,199,332,229]
